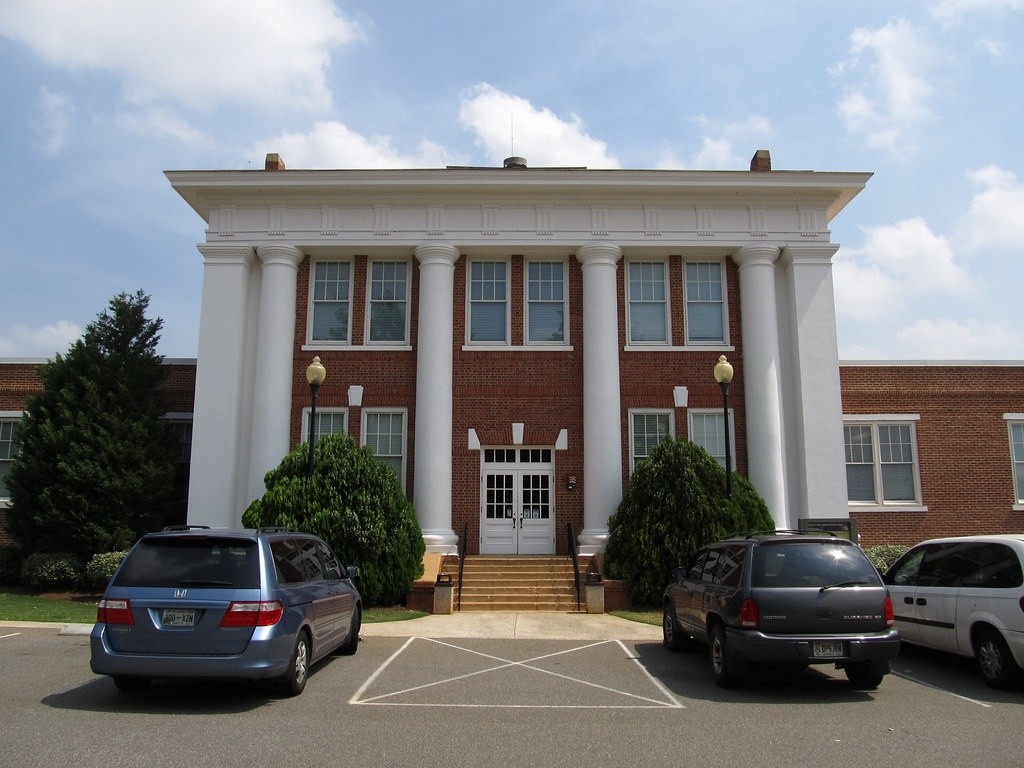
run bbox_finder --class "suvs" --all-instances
[89,524,364,696]
[662,528,901,690]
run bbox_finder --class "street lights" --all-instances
[306,354,326,480]
[713,354,734,503]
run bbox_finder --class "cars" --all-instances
[876,534,1024,694]
